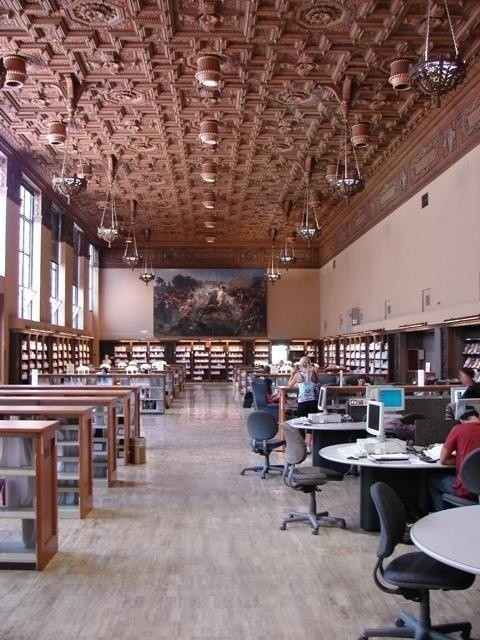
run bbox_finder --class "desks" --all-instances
[409,504,480,575]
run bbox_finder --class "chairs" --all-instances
[243,365,480,535]
[360,481,477,640]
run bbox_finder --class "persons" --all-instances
[427,405,480,511]
[41,368,178,452]
[236,357,480,454]
[153,277,265,336]
[100,354,112,366]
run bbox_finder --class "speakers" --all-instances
[419,349,424,360]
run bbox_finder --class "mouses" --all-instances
[304,423,312,425]
[346,456,359,460]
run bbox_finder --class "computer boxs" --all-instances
[357,439,406,454]
[308,413,346,422]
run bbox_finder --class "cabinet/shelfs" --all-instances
[98,340,247,380]
[1,418,62,573]
[1,329,184,520]
[253,317,480,393]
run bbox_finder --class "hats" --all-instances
[455,403,478,423]
[461,367,475,379]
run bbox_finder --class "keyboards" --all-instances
[338,444,366,458]
[296,417,306,423]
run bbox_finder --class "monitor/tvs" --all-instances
[376,388,405,411]
[318,387,329,414]
[451,387,468,403]
[366,401,384,443]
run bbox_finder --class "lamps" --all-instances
[188,45,229,248]
[263,39,467,289]
[2,42,155,292]
